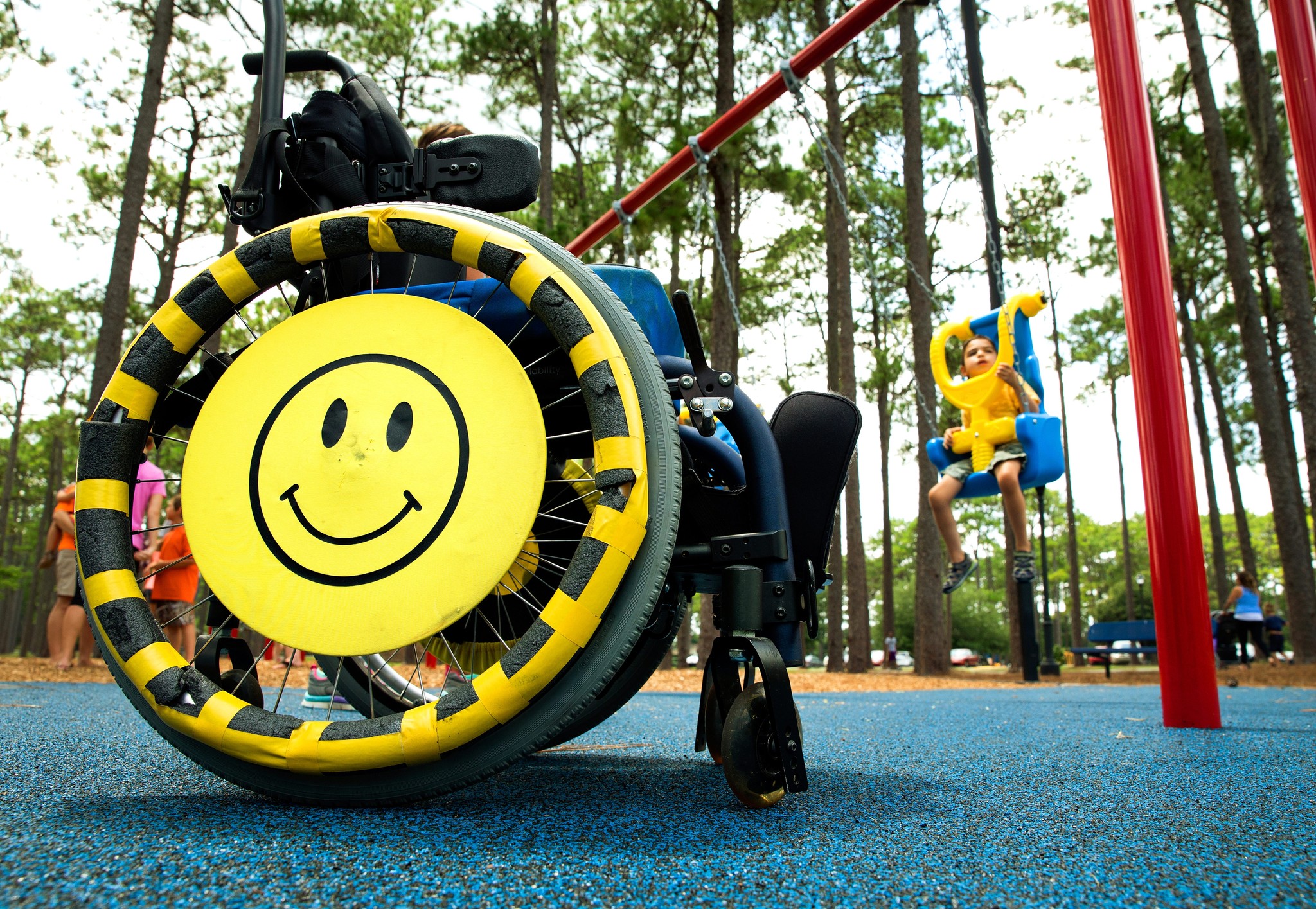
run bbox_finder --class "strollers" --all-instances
[1214,609,1241,661]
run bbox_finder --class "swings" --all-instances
[601,151,752,334]
[793,2,1067,501]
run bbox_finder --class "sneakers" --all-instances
[302,663,354,711]
[1010,550,1035,580]
[943,553,979,594]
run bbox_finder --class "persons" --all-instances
[1222,570,1292,669]
[883,630,898,666]
[418,123,490,281]
[39,428,478,711]
[927,335,1040,594]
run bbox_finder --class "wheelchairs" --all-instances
[70,49,864,811]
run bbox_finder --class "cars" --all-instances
[870,649,886,666]
[950,647,980,667]
[802,652,823,668]
[1088,639,1146,664]
[895,650,916,667]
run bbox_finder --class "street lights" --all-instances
[1133,571,1145,618]
